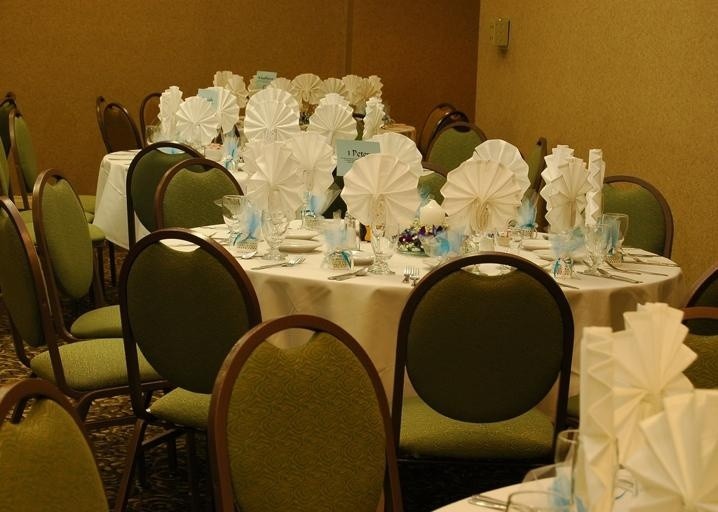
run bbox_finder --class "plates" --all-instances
[280,244,321,252]
[282,233,321,240]
[521,239,552,250]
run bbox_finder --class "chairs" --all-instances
[140,92,163,143]
[682,262,718,390]
[601,176,674,266]
[523,136,548,232]
[103,102,141,151]
[113,227,263,512]
[154,158,243,233]
[418,102,458,160]
[426,122,488,200]
[392,251,574,495]
[30,167,154,341]
[0,108,97,213]
[1,377,107,511]
[0,198,164,433]
[126,142,206,250]
[0,137,105,302]
[428,110,469,148]
[95,96,142,153]
[207,314,402,512]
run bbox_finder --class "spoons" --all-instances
[336,267,369,282]
[596,267,643,284]
[233,251,257,259]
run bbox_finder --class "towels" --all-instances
[289,74,324,105]
[351,78,379,109]
[198,87,240,135]
[611,389,718,511]
[340,153,422,237]
[244,99,300,144]
[343,75,363,92]
[245,88,300,118]
[318,93,353,115]
[468,139,531,201]
[360,97,385,141]
[440,157,522,235]
[317,77,348,99]
[267,78,291,90]
[213,71,249,109]
[158,86,184,135]
[586,149,606,224]
[367,75,384,99]
[306,103,358,151]
[248,75,262,98]
[175,97,220,147]
[366,132,423,179]
[246,143,307,218]
[285,131,336,194]
[545,156,593,233]
[614,301,698,466]
[576,326,618,512]
[540,144,574,210]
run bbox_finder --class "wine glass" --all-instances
[603,213,629,259]
[222,194,246,252]
[261,208,289,260]
[370,222,395,274]
[585,223,608,274]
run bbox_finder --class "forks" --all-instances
[403,266,411,283]
[250,257,305,270]
[632,257,681,267]
[410,266,420,286]
[604,260,668,276]
